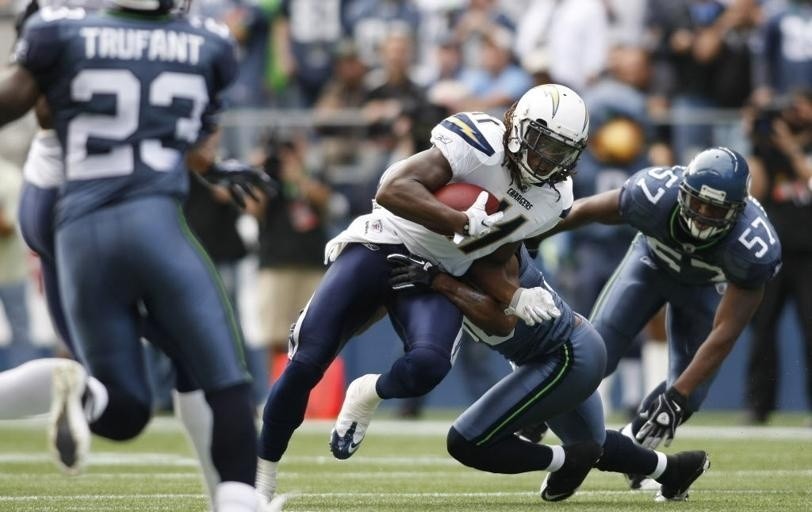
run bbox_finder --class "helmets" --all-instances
[108,0,192,16]
[675,143,752,243]
[505,83,590,186]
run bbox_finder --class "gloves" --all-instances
[385,252,438,298]
[200,156,279,210]
[635,387,691,451]
[503,285,562,327]
[453,191,504,246]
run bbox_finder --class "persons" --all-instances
[1,0,812,423]
[1,0,282,511]
[385,240,711,504]
[256,84,589,500]
[524,150,779,449]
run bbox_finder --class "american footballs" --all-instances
[433,184,501,215]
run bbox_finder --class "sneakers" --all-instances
[330,374,377,461]
[540,439,604,500]
[617,423,647,488]
[654,450,711,501]
[45,360,93,474]
